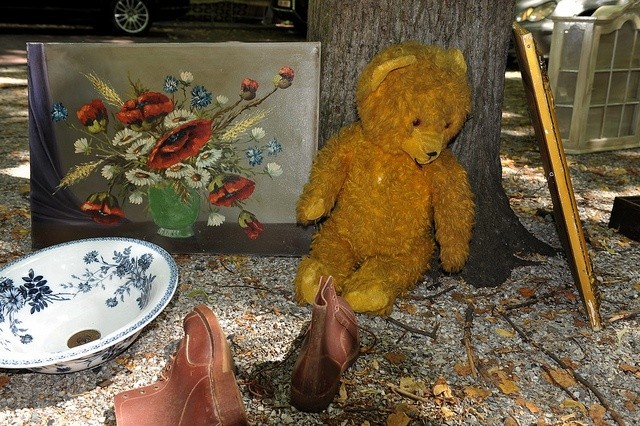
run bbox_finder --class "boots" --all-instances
[248,275,377,413]
[113,303,247,425]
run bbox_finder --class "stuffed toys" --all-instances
[295,43,477,318]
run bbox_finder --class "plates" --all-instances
[1,237,179,375]
